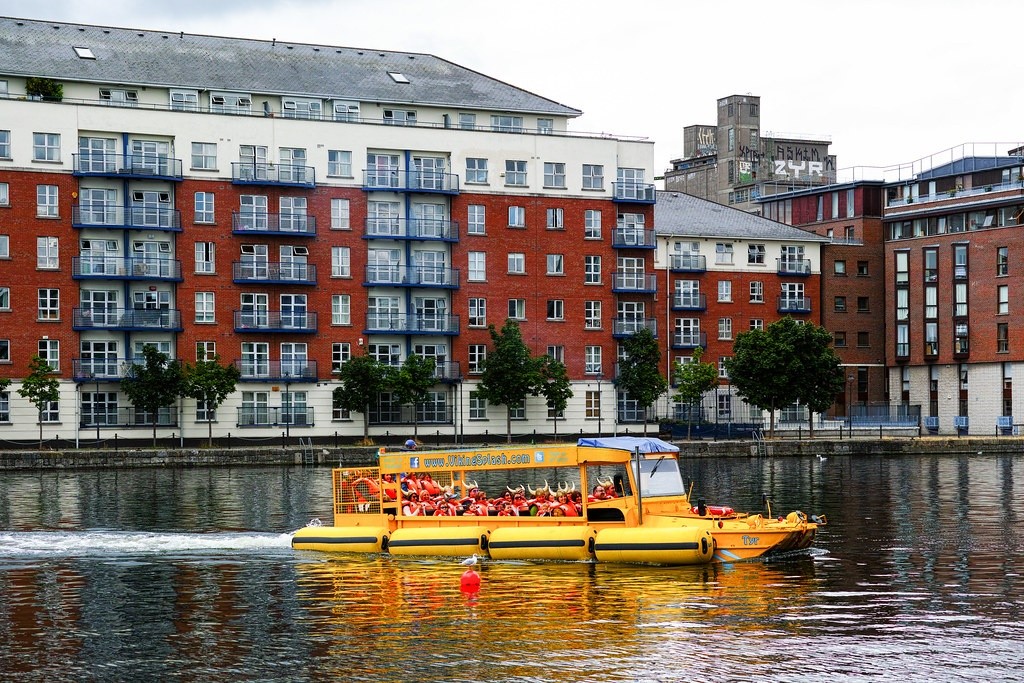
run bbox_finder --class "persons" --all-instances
[498,502,516,516]
[338,470,586,513]
[604,482,618,498]
[420,490,437,511]
[421,473,447,498]
[474,491,490,517]
[433,502,457,516]
[530,488,553,517]
[402,491,426,516]
[589,486,614,501]
[553,490,579,517]
[571,491,583,517]
[462,501,481,516]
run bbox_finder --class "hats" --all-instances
[444,492,457,501]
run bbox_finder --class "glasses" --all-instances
[506,494,510,497]
[412,496,417,498]
[440,506,447,509]
[472,489,479,493]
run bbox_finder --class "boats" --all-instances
[290,437,825,564]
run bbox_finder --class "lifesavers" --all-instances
[692,504,736,518]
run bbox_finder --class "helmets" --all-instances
[400,487,417,497]
[462,479,478,490]
[507,484,526,494]
[528,478,548,496]
[558,481,575,494]
[548,481,568,497]
[597,476,614,490]
[438,479,455,492]
[405,440,415,449]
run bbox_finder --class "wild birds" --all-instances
[460,553,479,570]
